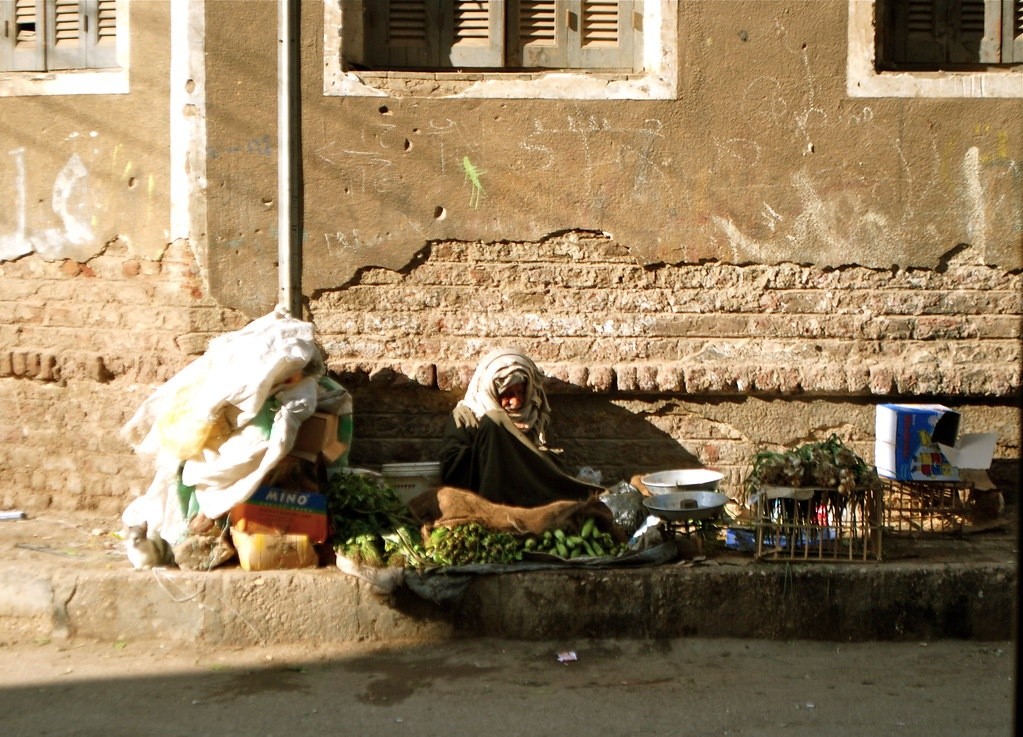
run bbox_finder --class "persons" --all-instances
[440,348,615,535]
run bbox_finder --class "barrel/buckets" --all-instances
[382,462,441,502]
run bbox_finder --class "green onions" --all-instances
[739,433,880,549]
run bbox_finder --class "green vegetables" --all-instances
[327,469,521,570]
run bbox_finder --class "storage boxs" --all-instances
[226,410,348,572]
[875,402,999,484]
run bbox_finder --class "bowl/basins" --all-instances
[641,470,725,495]
